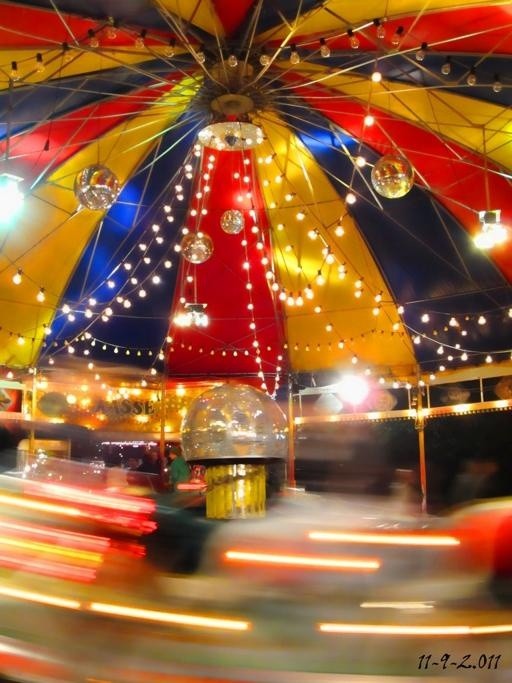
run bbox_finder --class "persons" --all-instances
[103,445,191,492]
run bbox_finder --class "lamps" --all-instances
[1,13,512,429]
